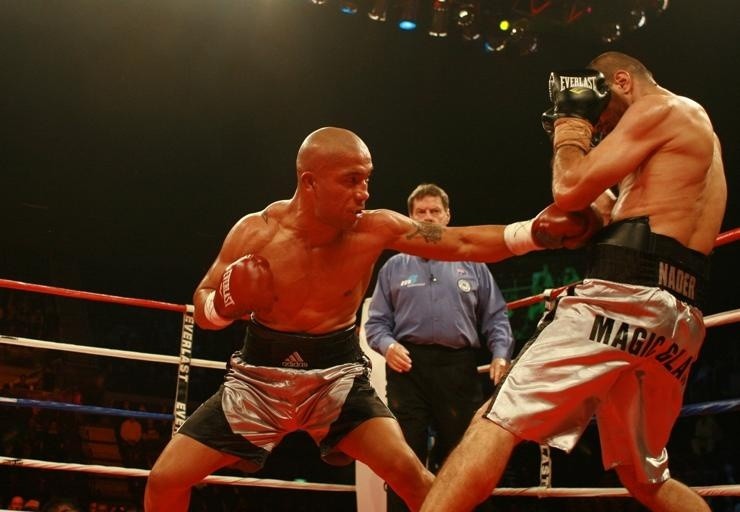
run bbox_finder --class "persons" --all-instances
[143,126,599,510]
[363,181,517,473]
[1,349,196,512]
[415,51,728,511]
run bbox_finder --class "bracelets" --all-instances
[204,292,234,327]
[504,216,540,257]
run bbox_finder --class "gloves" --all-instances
[212,252,280,319]
[530,198,602,253]
[542,66,614,149]
